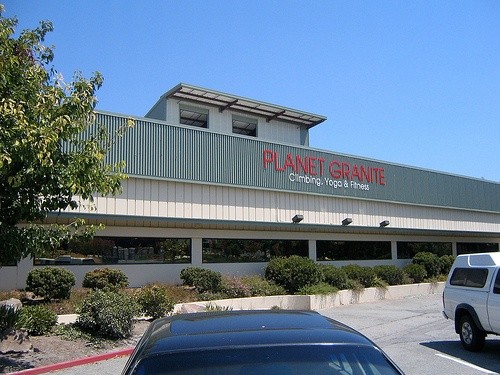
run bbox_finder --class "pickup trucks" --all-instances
[442,250,499,351]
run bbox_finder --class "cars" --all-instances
[119,310,407,375]
